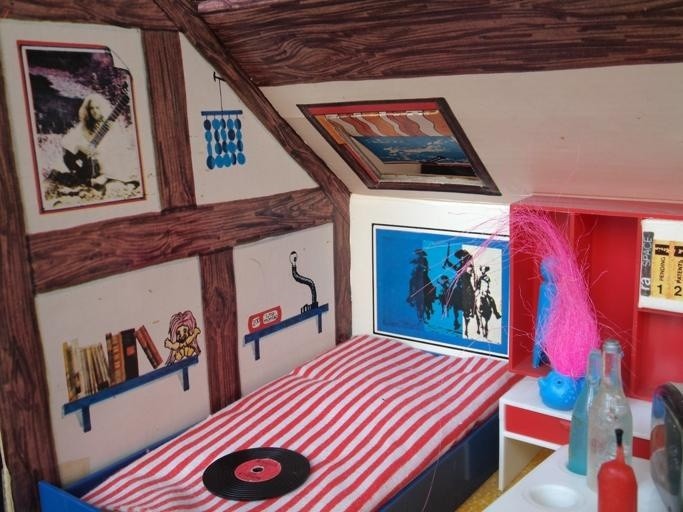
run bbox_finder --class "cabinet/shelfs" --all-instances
[499,194,683,491]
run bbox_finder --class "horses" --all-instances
[411,264,492,339]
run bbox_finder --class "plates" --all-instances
[522,483,598,511]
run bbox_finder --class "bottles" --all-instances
[568,339,635,493]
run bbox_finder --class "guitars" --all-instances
[63,81,130,182]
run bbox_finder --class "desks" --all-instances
[483,441,675,510]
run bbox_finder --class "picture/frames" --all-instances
[296,97,503,196]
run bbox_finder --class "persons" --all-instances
[61,92,126,191]
[407,249,501,319]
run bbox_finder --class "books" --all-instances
[62,325,163,402]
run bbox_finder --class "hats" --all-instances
[480,265,490,272]
[441,275,448,279]
[454,249,473,259]
[416,250,427,256]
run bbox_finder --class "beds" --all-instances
[39,333,524,511]
